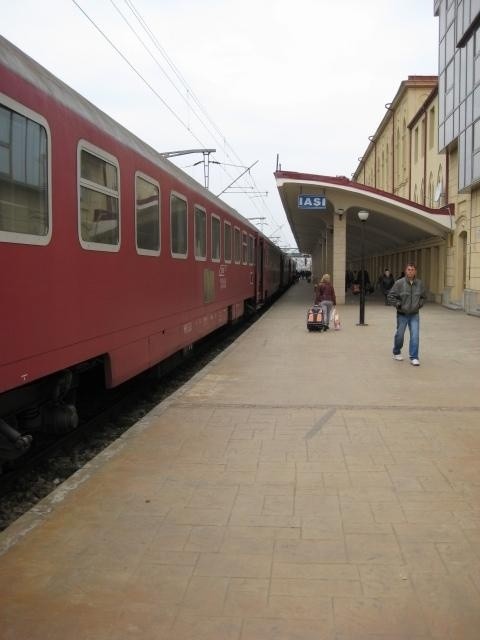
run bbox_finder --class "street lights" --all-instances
[353,206,370,326]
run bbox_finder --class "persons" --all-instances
[290,268,370,297]
[378,268,395,306]
[387,262,426,366]
[315,273,336,331]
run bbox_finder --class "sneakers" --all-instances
[411,359,419,365]
[393,355,403,360]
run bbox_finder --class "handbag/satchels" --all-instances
[334,311,340,330]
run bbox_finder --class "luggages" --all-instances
[307,305,324,331]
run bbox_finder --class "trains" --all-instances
[0,34,298,467]
